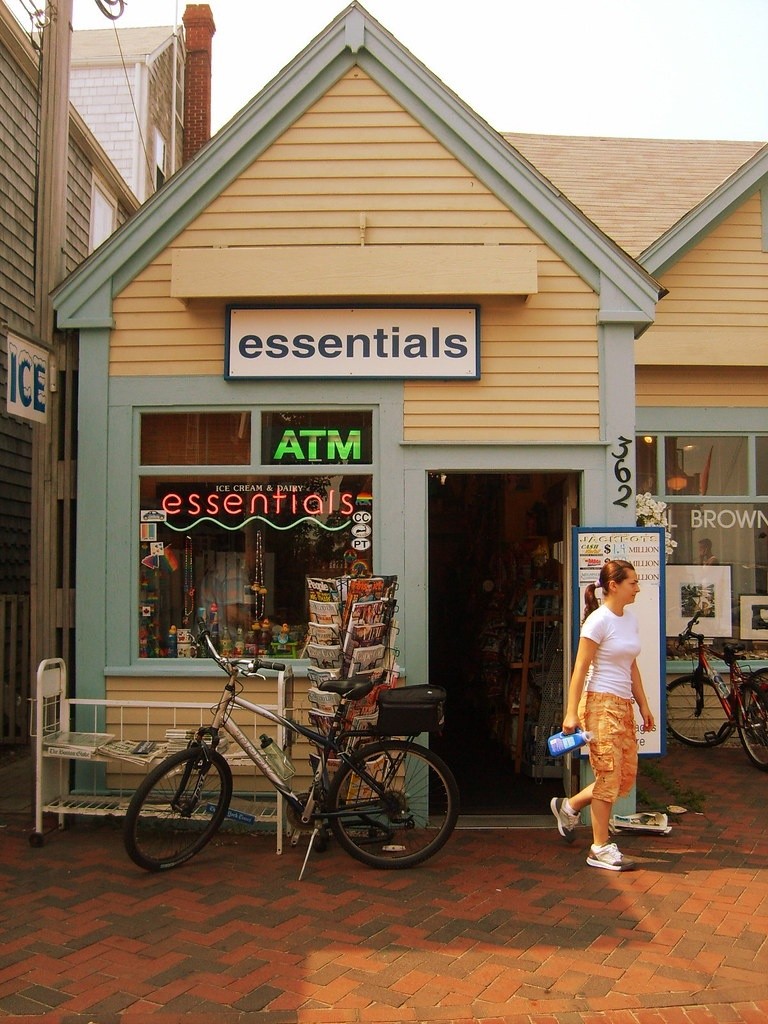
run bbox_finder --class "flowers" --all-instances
[635,492,678,565]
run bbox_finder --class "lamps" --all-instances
[667,448,688,496]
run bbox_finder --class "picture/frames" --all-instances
[738,592,768,642]
[664,562,734,640]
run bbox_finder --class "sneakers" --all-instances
[550,798,581,843]
[587,843,636,871]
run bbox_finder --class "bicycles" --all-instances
[665,606,768,770]
[121,627,460,882]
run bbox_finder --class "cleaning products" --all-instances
[548,728,594,756]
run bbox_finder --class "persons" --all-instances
[696,539,718,581]
[187,531,274,657]
[550,560,654,871]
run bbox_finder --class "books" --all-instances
[306,577,399,805]
[608,811,673,836]
[118,793,265,825]
[43,728,267,767]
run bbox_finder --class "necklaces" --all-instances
[184,536,194,616]
[251,530,268,621]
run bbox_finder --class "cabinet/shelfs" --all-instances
[507,589,563,785]
[28,658,294,857]
